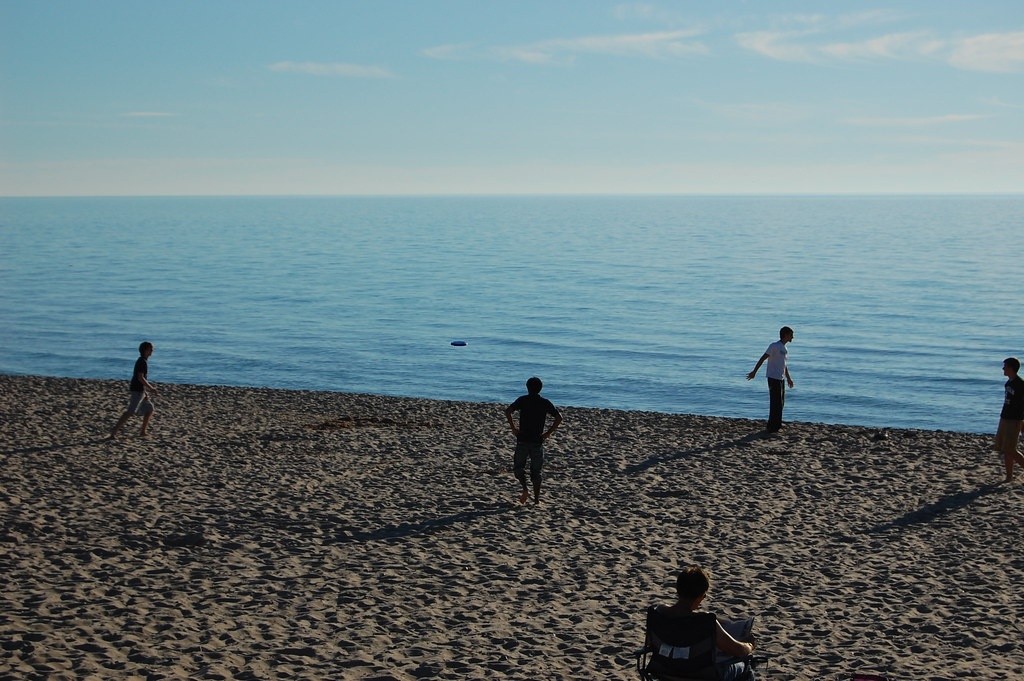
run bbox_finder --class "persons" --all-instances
[110,342,158,439]
[995,358,1024,484]
[645,568,754,681]
[506,378,563,504]
[746,327,793,433]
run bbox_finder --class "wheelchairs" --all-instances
[633,605,757,681]
